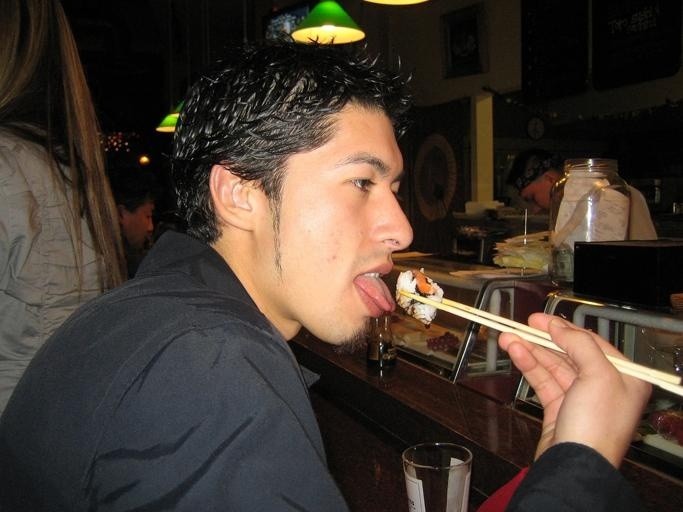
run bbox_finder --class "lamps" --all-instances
[293,0,364,45]
[155,102,181,133]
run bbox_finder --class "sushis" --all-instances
[395,269,444,325]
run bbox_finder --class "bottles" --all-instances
[548,157,635,287]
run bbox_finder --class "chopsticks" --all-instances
[400,287,683,396]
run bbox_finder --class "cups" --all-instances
[366,314,398,370]
[401,440,472,510]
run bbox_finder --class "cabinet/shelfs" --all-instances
[286,244,683,511]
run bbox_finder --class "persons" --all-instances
[506,147,657,239]
[110,170,156,276]
[1,40,651,509]
[1,0,128,410]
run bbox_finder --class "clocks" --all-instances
[523,116,546,141]
